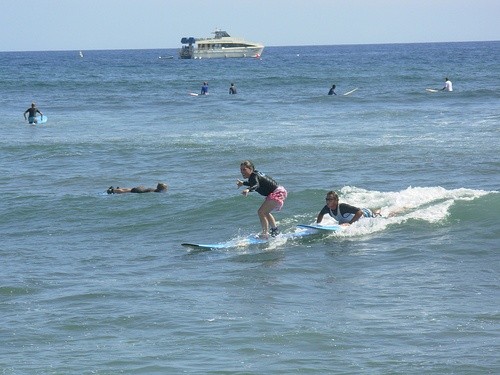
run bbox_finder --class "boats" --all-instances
[179,30,265,60]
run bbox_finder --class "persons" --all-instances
[439,78,452,92]
[201,82,209,94]
[229,83,237,94]
[108,183,168,194]
[329,85,338,95]
[316,191,381,227]
[24,103,43,124]
[236,161,287,235]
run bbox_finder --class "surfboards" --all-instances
[296,223,345,231]
[181,232,284,249]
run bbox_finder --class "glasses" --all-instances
[326,198,334,201]
